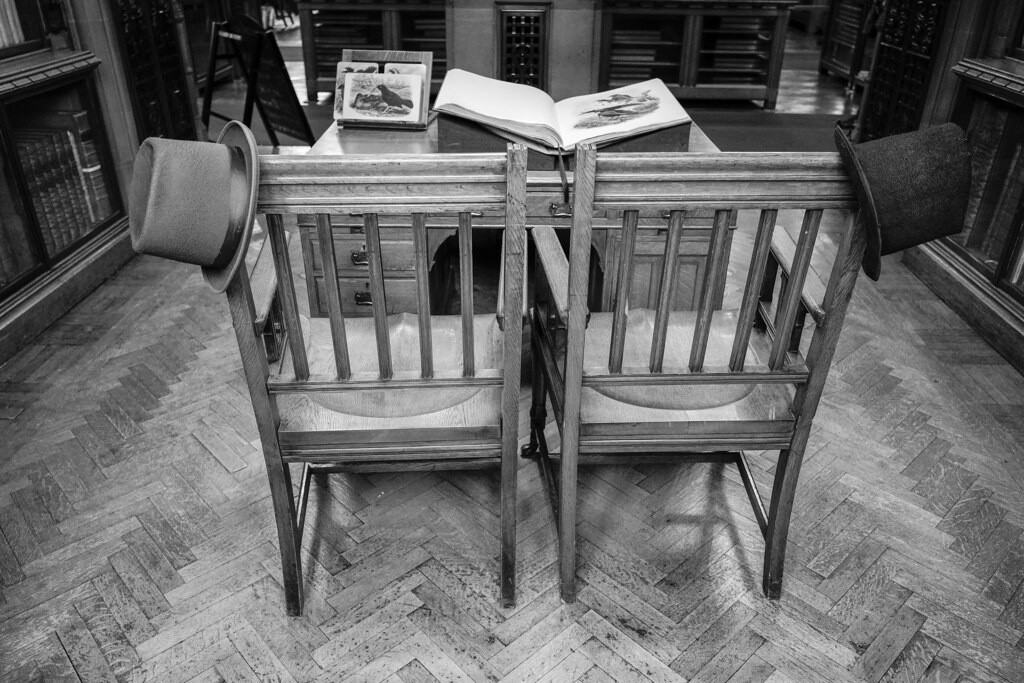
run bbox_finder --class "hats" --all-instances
[128,120,260,293]
[834,120,973,282]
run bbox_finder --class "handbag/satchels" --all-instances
[429,224,603,318]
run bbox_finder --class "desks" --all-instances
[300,104,723,319]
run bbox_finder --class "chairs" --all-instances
[528,162,882,602]
[220,140,534,616]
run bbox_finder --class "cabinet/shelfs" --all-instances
[297,1,794,111]
[854,0,1024,365]
[1,0,203,361]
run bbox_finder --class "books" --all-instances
[432,69,692,156]
[0,90,111,288]
[0,0,25,50]
